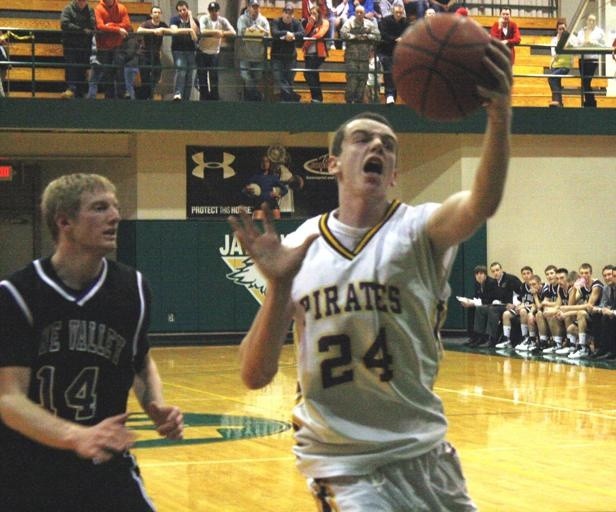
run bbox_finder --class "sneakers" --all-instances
[462,336,494,349]
[62,89,75,99]
[515,336,578,355]
[496,337,513,348]
[587,349,616,364]
[568,345,588,360]
[174,94,182,100]
[386,95,394,103]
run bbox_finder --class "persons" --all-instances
[491,6,522,66]
[1,173,185,511]
[577,14,606,107]
[61,1,469,107]
[546,21,577,106]
[0,31,11,97]
[242,148,303,224]
[228,37,515,512]
[612,38,616,61]
[457,263,616,362]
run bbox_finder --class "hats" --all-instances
[248,0,259,6]
[209,2,221,9]
[455,7,468,16]
[283,4,294,10]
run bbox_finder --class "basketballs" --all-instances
[392,13,497,121]
